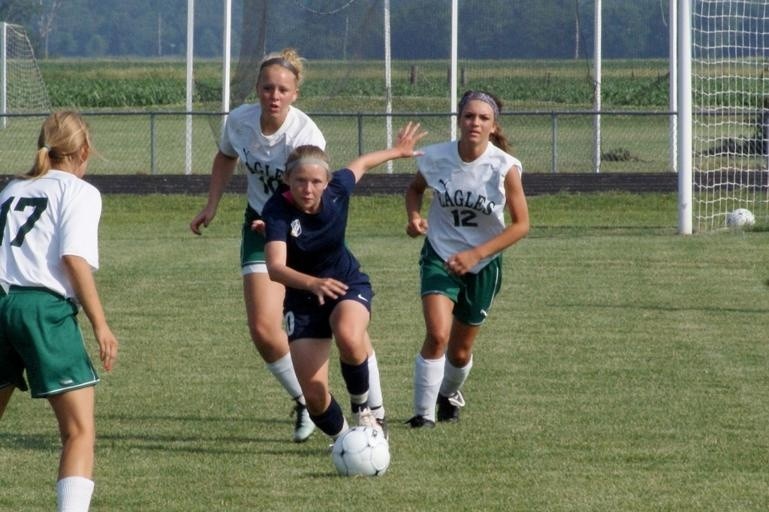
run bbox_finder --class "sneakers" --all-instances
[291,401,320,443]
[349,391,465,440]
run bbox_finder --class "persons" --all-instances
[262,119,428,447]
[189,49,328,442]
[1,109,119,512]
[405,89,532,425]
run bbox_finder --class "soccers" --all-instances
[727,209,755,227]
[332,428,391,477]
